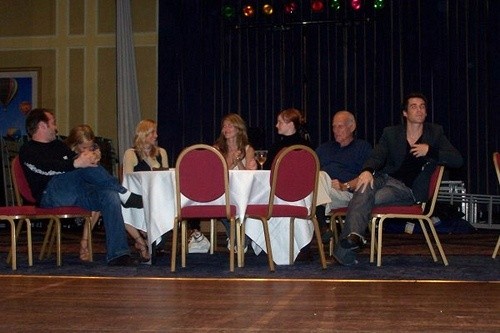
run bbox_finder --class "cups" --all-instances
[79,144,100,168]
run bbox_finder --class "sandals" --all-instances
[79,239,89,260]
[136,241,149,262]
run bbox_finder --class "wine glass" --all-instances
[253,151,268,170]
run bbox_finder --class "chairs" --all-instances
[240,144,328,271]
[492,152,500,258]
[0,153,93,270]
[369,165,449,268]
[329,206,379,257]
[171,145,243,272]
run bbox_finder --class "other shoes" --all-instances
[236,245,248,252]
[322,232,334,243]
[109,257,137,267]
[341,235,364,254]
[121,192,143,209]
[344,252,357,265]
[227,237,235,252]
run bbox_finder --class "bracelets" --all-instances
[236,157,240,161]
[344,182,351,189]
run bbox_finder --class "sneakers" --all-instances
[193,230,203,242]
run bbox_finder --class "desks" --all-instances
[122,170,332,266]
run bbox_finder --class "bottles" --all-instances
[404,222,415,234]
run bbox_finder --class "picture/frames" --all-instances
[0,66,42,139]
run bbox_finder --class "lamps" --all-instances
[223,0,388,31]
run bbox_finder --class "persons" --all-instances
[123,119,212,253]
[19,108,144,265]
[65,125,151,260]
[251,108,314,256]
[212,113,257,253]
[295,110,376,262]
[320,93,464,266]
